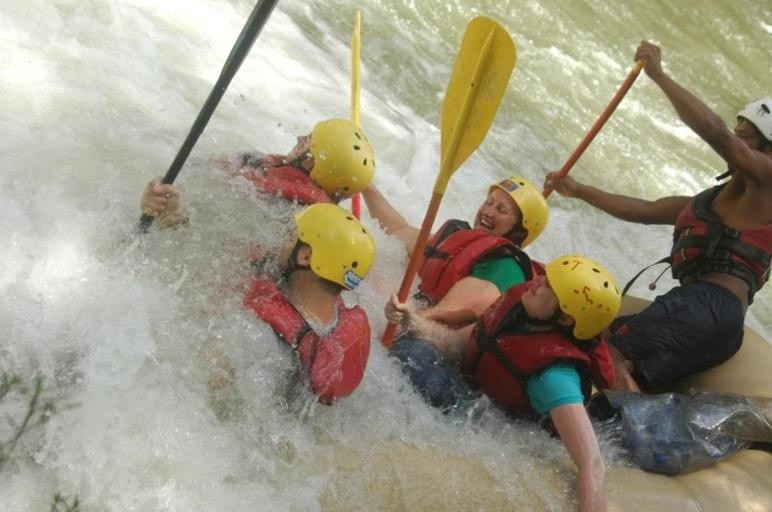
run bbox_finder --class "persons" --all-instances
[215,117,380,208]
[542,37,772,473]
[383,254,623,512]
[139,177,377,421]
[361,175,550,329]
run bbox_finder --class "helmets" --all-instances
[545,255,623,341]
[309,118,375,200]
[295,203,375,292]
[737,98,771,140]
[490,175,551,252]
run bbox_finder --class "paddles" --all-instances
[351,10,360,222]
[382,18,516,347]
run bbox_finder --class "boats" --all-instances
[290,276,772,512]
